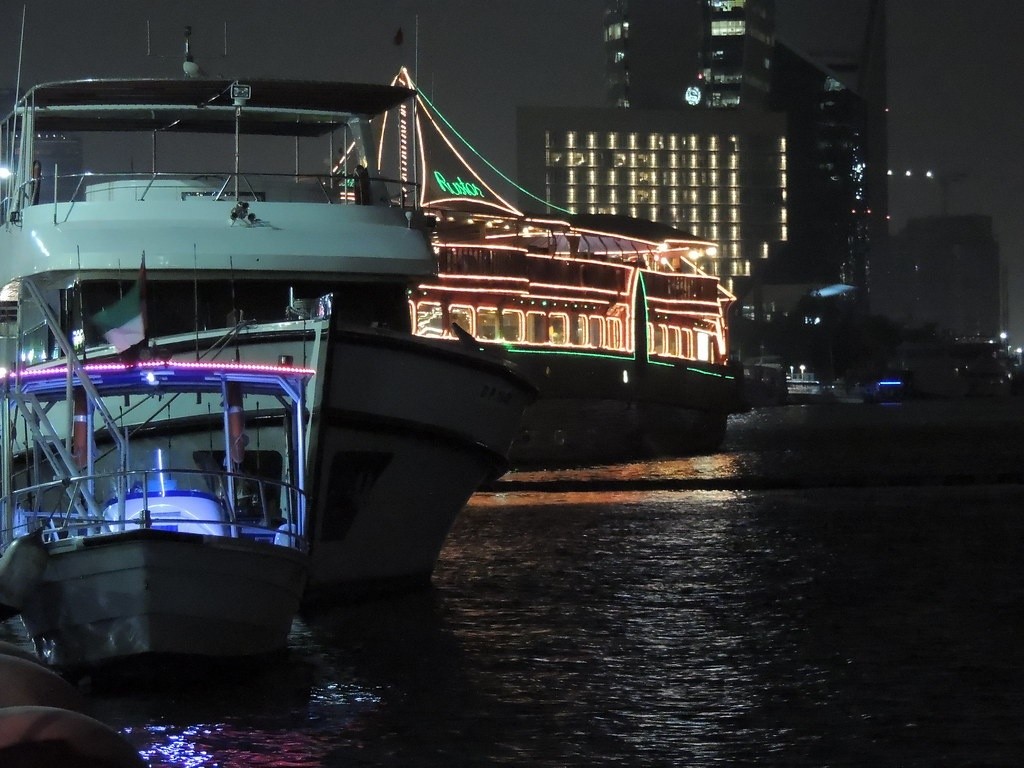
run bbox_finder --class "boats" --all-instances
[1,363,320,675]
[785,363,823,405]
[323,62,749,469]
[0,0,542,611]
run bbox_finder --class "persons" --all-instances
[1,641,149,768]
[355,165,369,205]
